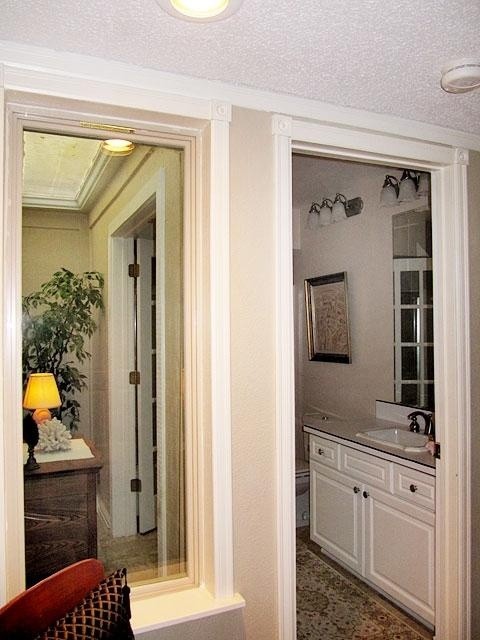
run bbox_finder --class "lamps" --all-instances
[307,195,362,230]
[23,372,63,423]
[377,170,428,209]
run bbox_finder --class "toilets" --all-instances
[295,410,345,528]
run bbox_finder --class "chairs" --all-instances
[1,558,103,639]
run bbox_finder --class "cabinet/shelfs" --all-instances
[25,437,104,588]
[303,426,436,633]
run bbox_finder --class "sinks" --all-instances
[355,425,433,453]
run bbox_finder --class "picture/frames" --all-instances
[303,270,353,365]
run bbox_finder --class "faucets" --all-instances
[407,410,433,436]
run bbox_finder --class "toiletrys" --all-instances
[425,434,435,456]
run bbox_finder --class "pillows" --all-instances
[33,569,131,640]
